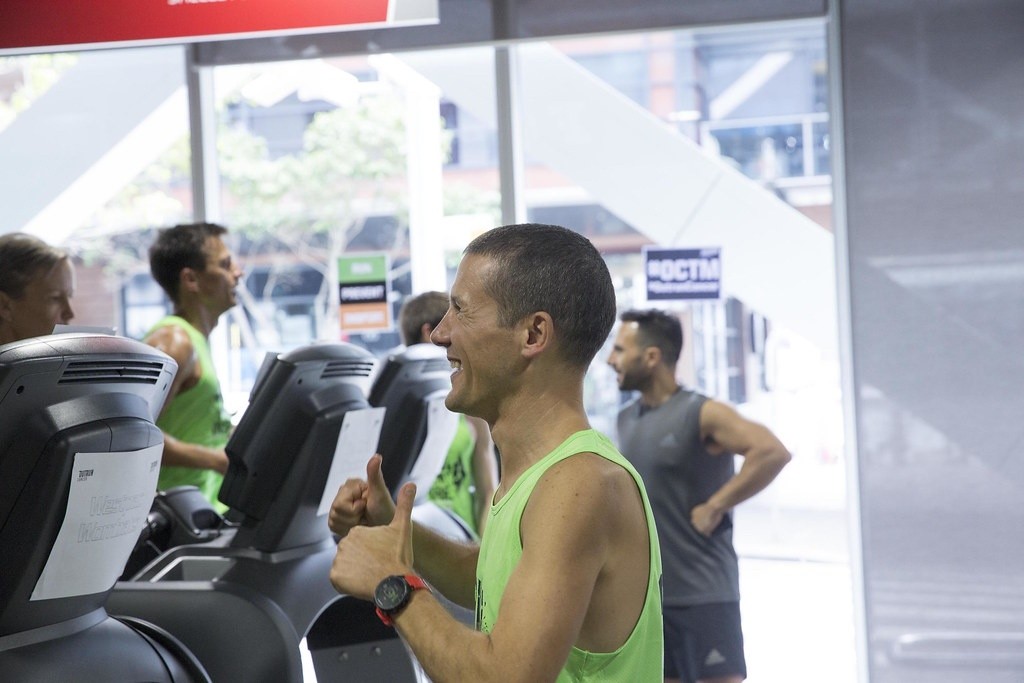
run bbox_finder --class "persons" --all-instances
[328,225,666,683]
[0,231,75,345]
[144,224,244,512]
[608,309,792,683]
[401,291,500,538]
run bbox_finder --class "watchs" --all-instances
[374,574,433,626]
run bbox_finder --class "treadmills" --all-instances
[1,330,482,683]
